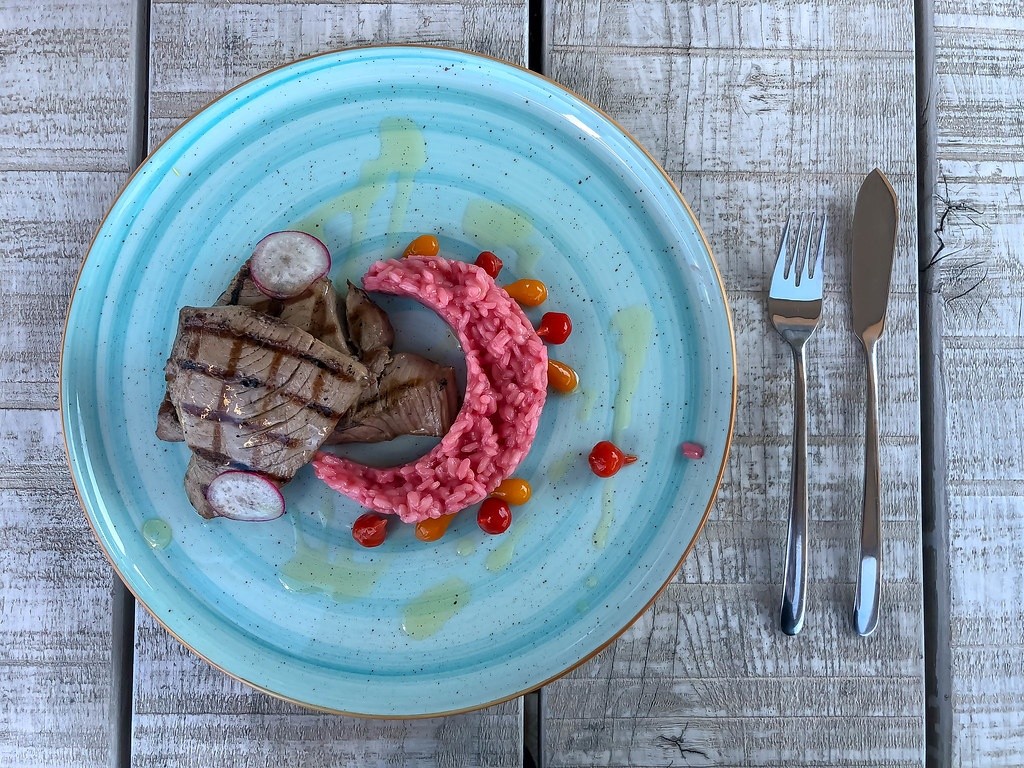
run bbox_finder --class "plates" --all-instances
[59,45,737,718]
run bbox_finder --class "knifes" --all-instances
[851,168,907,636]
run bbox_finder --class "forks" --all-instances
[767,211,827,636]
